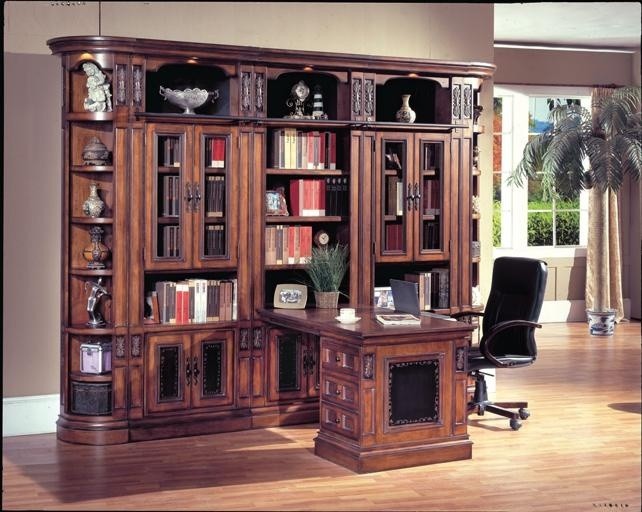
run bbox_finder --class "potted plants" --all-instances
[300,241,350,308]
[507,84,641,336]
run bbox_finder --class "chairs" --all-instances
[449,256,547,430]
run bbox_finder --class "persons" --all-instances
[83,278,108,323]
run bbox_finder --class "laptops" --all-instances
[389,279,450,319]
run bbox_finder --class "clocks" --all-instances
[315,231,329,249]
[319,233,329,245]
[295,82,309,101]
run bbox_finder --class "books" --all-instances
[144,128,452,329]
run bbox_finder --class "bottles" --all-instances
[83,226,109,268]
[82,182,104,217]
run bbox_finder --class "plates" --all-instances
[334,316,362,323]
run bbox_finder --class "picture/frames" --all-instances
[264,187,289,217]
[272,285,310,309]
[374,287,397,312]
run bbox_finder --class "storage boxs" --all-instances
[79,340,111,375]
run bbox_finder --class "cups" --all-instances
[340,308,356,319]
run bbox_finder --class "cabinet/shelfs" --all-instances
[319,337,360,448]
[268,327,320,401]
[144,120,239,274]
[373,132,451,265]
[63,51,111,421]
[145,330,233,415]
[119,36,474,127]
[264,168,351,273]
[472,85,483,316]
